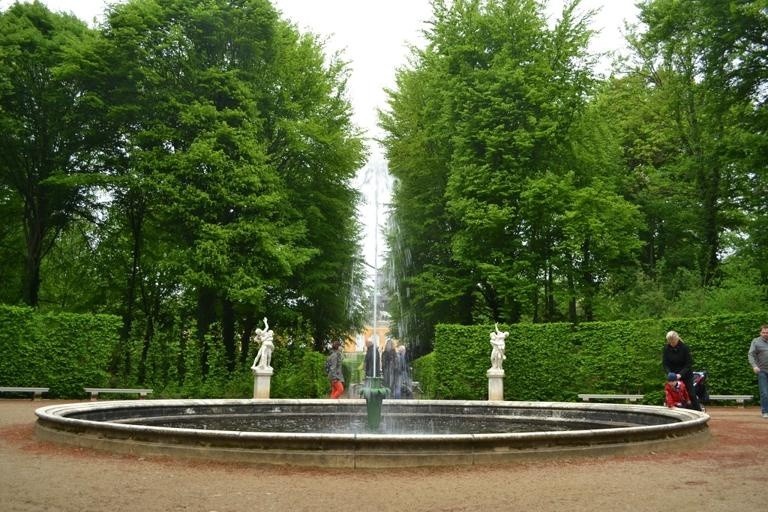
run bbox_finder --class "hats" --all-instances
[667,372,681,382]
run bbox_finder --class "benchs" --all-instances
[576,390,646,404]
[0,386,50,400]
[710,394,754,409]
[83,386,154,401]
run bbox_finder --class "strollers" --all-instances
[676,371,708,412]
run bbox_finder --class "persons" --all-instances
[251,316,275,369]
[664,371,691,409]
[395,344,413,399]
[326,341,345,399]
[748,324,768,419]
[489,321,510,370]
[661,331,702,411]
[364,338,381,377]
[382,338,400,399]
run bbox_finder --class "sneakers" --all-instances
[760,409,768,418]
